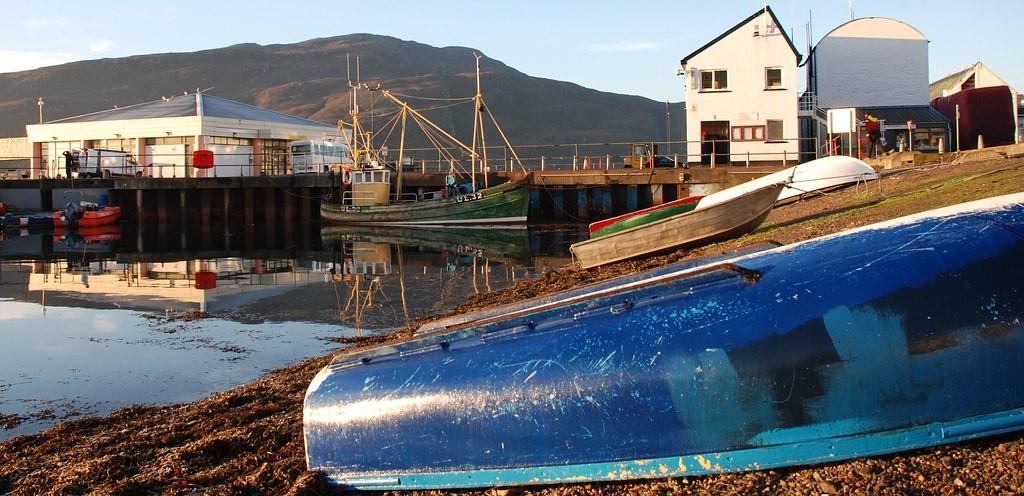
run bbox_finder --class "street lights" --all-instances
[37,97,45,124]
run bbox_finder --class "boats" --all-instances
[589,196,706,238]
[0,202,54,228]
[316,51,533,223]
[571,179,787,271]
[694,154,880,209]
[319,222,534,339]
[52,201,121,227]
[52,223,122,244]
[304,192,1024,492]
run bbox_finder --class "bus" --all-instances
[289,139,355,176]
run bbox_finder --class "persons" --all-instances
[65,263,90,289]
[864,112,887,160]
[63,151,74,179]
[77,148,90,179]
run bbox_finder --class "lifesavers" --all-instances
[343,257,354,274]
[342,170,352,186]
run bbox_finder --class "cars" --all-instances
[390,149,414,166]
[654,155,683,167]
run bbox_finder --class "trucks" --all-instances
[70,147,144,179]
